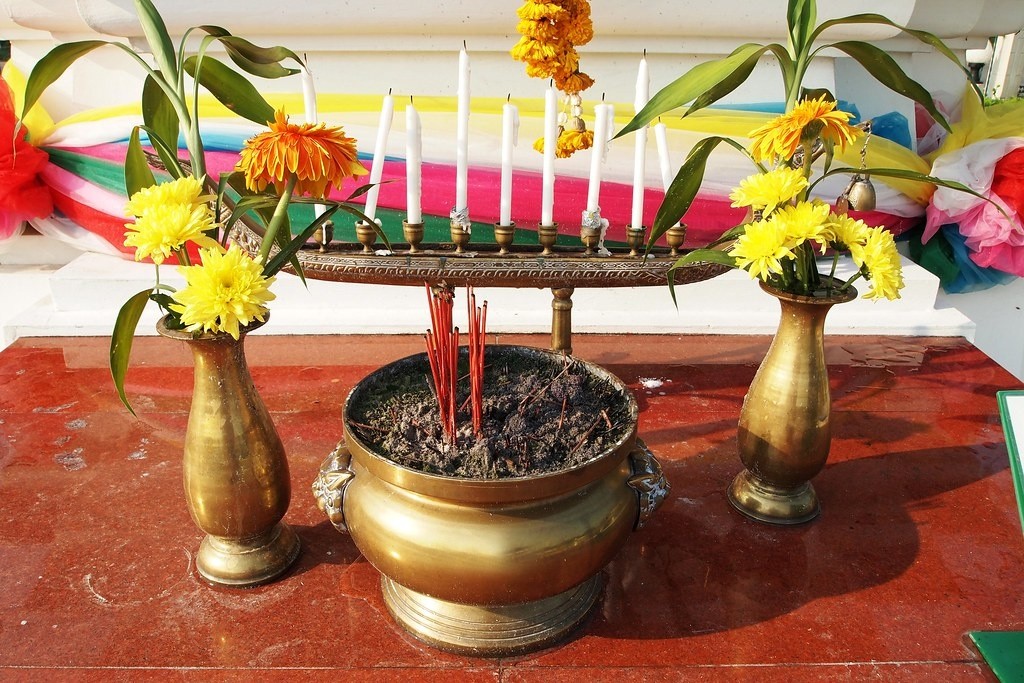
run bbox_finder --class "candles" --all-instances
[278,41,685,260]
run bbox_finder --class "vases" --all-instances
[722,271,859,528]
[156,306,304,590]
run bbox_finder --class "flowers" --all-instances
[11,0,395,417]
[607,2,1012,308]
[513,0,597,159]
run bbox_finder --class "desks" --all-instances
[1,334,1024,681]
